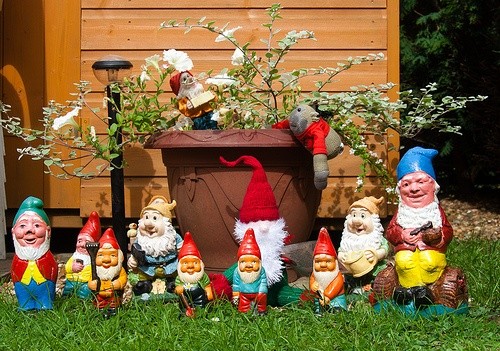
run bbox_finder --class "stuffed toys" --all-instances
[271,103,341,190]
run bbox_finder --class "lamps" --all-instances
[91,54,134,86]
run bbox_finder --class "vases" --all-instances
[144,128,321,283]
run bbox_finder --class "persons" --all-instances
[11,196,58,313]
[169,70,219,130]
[62,146,454,319]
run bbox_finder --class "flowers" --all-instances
[0,2,488,205]
